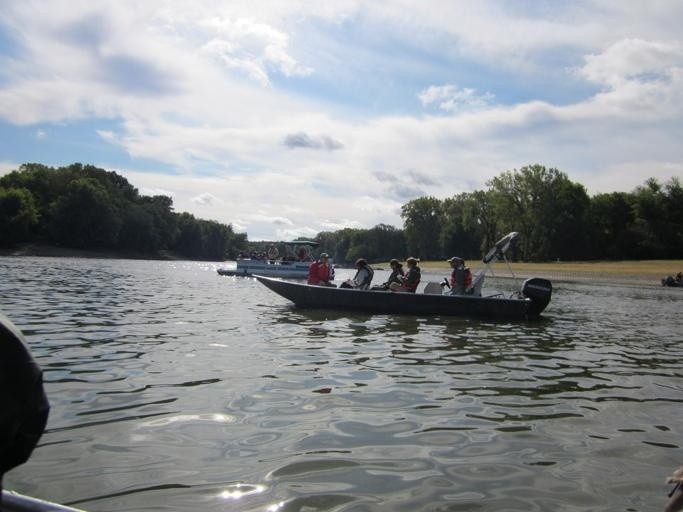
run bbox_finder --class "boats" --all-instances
[661,271,683,290]
[254,232,556,329]
[215,238,338,283]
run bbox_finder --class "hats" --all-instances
[390,259,399,265]
[321,253,327,257]
[448,257,464,265]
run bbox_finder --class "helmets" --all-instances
[356,258,367,265]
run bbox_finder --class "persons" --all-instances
[307,252,337,287]
[268,243,279,264]
[371,257,421,292]
[444,257,472,297]
[237,251,243,260]
[339,258,374,290]
[251,251,267,259]
